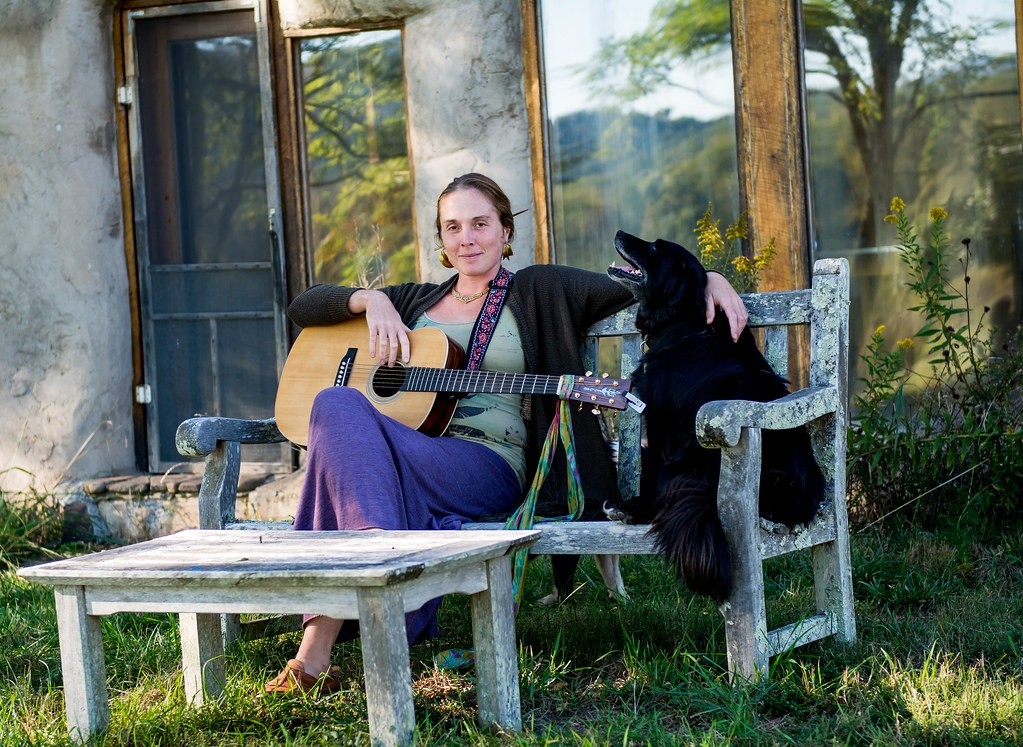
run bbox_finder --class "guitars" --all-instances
[275,314,632,452]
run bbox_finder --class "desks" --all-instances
[16,528,543,747]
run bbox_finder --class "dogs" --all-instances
[603,230,828,610]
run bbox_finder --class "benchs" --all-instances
[173,258,858,687]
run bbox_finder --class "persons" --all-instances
[263,173,749,696]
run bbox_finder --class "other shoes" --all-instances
[266,659,340,694]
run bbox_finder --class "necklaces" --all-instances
[452,288,490,303]
